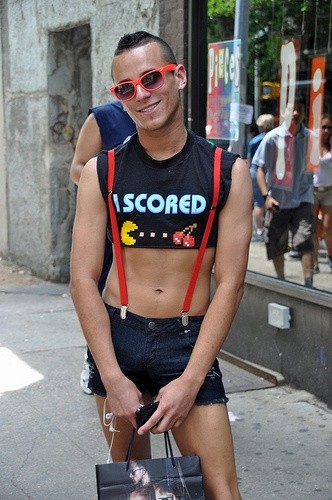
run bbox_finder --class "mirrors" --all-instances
[184,0,332,311]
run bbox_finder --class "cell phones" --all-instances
[136,401,164,431]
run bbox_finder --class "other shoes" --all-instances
[314,255,332,274]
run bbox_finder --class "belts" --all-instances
[313,186,332,193]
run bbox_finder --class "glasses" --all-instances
[110,64,180,102]
[322,125,332,129]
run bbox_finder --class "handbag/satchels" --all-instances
[95,425,205,500]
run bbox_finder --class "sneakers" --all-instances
[80,353,93,395]
[251,230,266,242]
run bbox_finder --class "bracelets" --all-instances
[262,194,269,199]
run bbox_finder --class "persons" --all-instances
[128,461,177,500]
[70,30,253,500]
[248,95,332,290]
